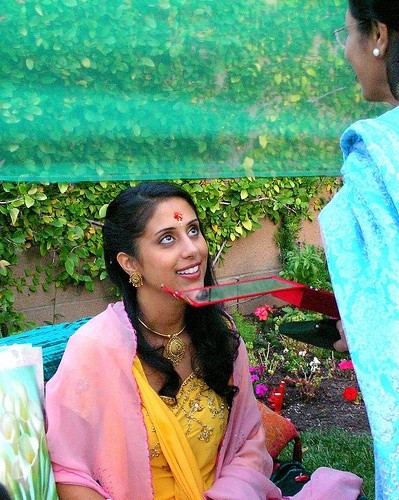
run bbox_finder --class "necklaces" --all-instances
[136,315,190,365]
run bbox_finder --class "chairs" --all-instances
[0,318,315,496]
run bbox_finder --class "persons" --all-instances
[318,0,399,499]
[43,181,286,499]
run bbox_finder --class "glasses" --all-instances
[333,18,379,53]
